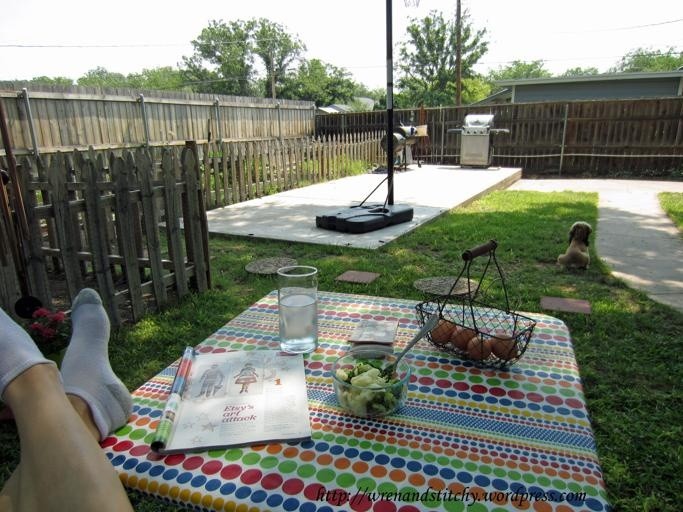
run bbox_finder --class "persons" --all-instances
[193,363,226,399]
[0,282,141,510]
[232,362,259,393]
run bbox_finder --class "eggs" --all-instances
[430,319,519,360]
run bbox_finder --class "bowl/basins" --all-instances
[330,349,411,418]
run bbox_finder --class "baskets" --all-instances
[415,294,537,371]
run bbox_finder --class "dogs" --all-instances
[557,220,592,269]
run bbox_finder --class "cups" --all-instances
[274,264,320,355]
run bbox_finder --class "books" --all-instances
[150,345,312,456]
